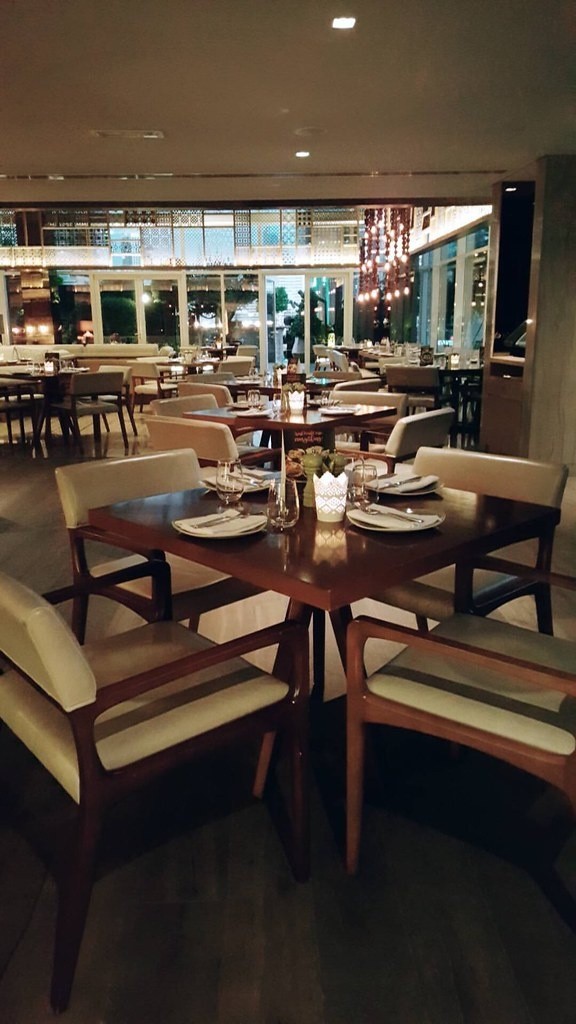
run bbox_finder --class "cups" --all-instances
[216,457,244,502]
[349,465,380,509]
[247,389,261,410]
[267,477,299,528]
[320,389,336,408]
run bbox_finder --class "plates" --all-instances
[171,510,268,539]
[203,469,275,492]
[226,401,264,409]
[317,408,355,415]
[305,398,340,408]
[347,505,446,533]
[237,409,270,417]
[366,474,443,495]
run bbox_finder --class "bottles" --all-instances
[302,453,322,507]
[327,454,347,478]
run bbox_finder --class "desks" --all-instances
[154,357,220,375]
[89,459,559,800]
[438,361,484,448]
[181,401,397,469]
[207,378,348,409]
[1,363,87,453]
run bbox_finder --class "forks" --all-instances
[353,500,423,524]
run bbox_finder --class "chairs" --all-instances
[0,337,576,1015]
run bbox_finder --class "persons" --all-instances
[282,316,295,362]
